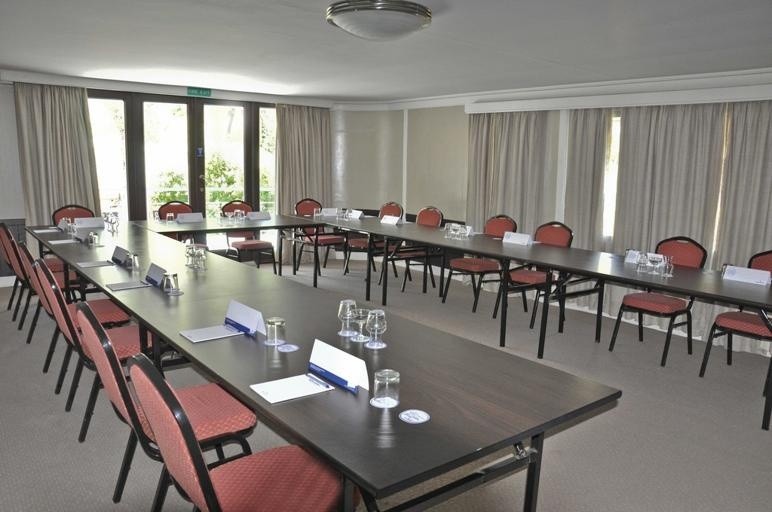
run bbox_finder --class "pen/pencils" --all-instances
[140,280,148,285]
[306,373,329,388]
[107,260,113,264]
[226,324,240,332]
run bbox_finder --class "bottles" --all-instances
[663,256,674,277]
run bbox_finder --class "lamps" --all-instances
[325,0,432,42]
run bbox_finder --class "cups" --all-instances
[126,253,139,269]
[313,207,321,216]
[68,223,77,235]
[267,318,286,344]
[374,368,399,407]
[88,231,98,244]
[163,273,178,291]
[166,213,175,223]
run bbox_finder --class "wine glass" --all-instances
[223,209,246,225]
[336,208,352,222]
[336,299,357,337]
[186,246,207,270]
[350,309,369,342]
[635,253,649,273]
[365,310,388,349]
[649,254,662,275]
[444,223,468,240]
[103,212,118,232]
